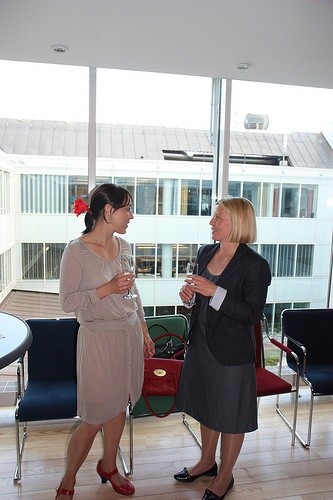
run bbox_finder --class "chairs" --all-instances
[254,313,300,447]
[13,318,84,482]
[117,314,202,476]
[276,308,333,449]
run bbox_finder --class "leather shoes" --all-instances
[201,474,234,500]
[174,462,217,483]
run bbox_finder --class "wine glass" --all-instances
[183,263,198,305]
[121,256,138,299]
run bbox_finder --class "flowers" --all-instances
[74,198,93,218]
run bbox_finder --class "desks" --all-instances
[0,311,34,371]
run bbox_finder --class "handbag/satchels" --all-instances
[142,324,187,418]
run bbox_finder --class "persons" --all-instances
[56,183,155,500]
[174,197,271,500]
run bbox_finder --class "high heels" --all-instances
[96,459,135,495]
[55,477,76,500]
